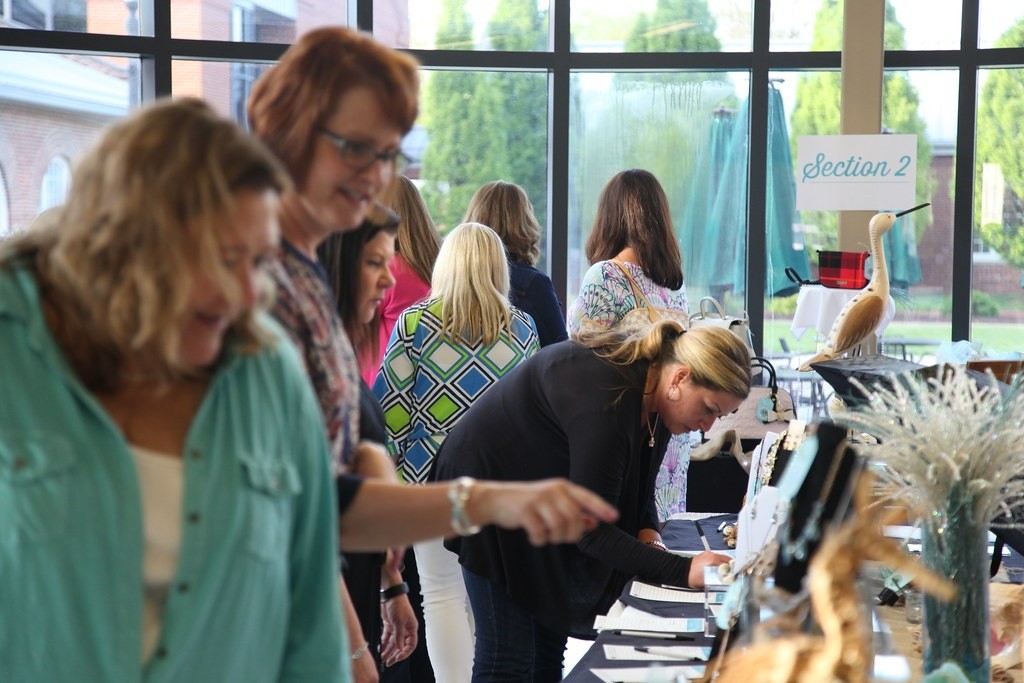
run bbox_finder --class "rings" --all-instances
[388,640,395,643]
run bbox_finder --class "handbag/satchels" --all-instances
[687,295,763,376]
[814,249,873,289]
[581,257,692,339]
[700,356,797,451]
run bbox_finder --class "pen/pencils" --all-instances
[717,521,726,532]
[694,520,711,550]
[634,646,703,661]
[611,630,696,640]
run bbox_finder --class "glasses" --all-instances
[315,125,411,175]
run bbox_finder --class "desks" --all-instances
[768,368,831,423]
[879,338,941,360]
[562,513,1024,683]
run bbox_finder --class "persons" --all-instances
[0,97,351,683]
[247,24,755,683]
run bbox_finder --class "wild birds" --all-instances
[796,203,932,374]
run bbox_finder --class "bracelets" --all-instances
[380,582,409,603]
[447,475,481,537]
[351,641,369,660]
[646,540,666,551]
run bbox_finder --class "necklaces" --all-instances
[644,364,659,447]
